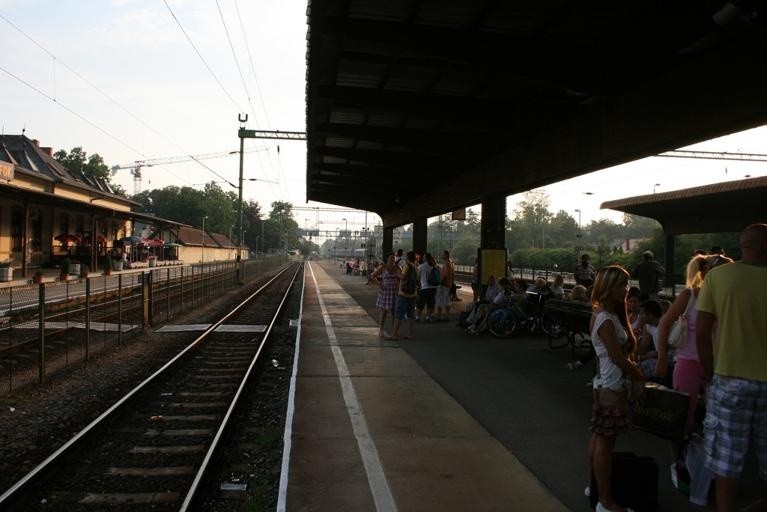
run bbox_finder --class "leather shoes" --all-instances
[596,502,633,512]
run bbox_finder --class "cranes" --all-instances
[112,149,240,196]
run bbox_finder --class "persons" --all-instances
[459,223,766,511]
[340,249,464,340]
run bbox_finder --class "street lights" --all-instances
[255,236,260,258]
[575,209,581,265]
[342,219,347,262]
[239,178,256,259]
[229,224,235,259]
[334,228,339,264]
[243,230,246,259]
[202,216,208,264]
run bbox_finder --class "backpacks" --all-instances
[401,264,417,294]
[428,264,440,285]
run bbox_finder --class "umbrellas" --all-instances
[53,233,183,261]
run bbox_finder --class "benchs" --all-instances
[539,297,595,356]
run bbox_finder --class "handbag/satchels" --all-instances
[667,287,694,347]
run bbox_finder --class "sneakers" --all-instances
[415,316,449,322]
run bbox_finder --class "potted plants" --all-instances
[1,253,158,284]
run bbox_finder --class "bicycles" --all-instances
[486,287,568,338]
